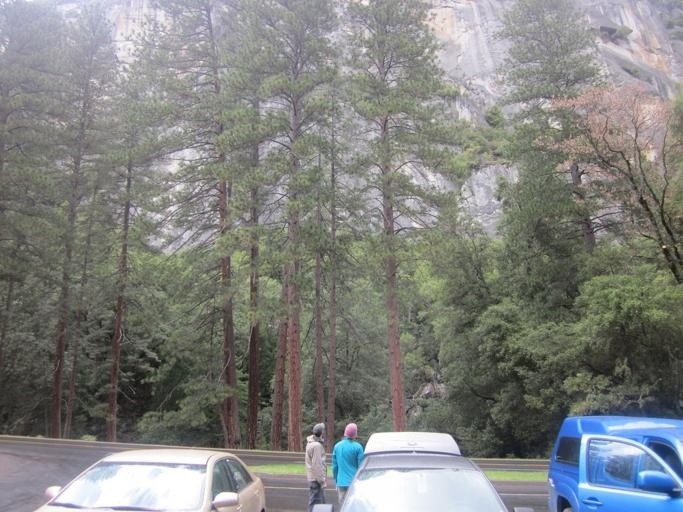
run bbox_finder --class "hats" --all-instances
[313,422,326,434]
[343,422,357,437]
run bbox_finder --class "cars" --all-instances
[34,448,267,511]
[309,432,536,512]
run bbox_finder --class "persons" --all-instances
[304,422,329,512]
[331,422,363,506]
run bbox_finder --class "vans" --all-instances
[547,415,683,511]
